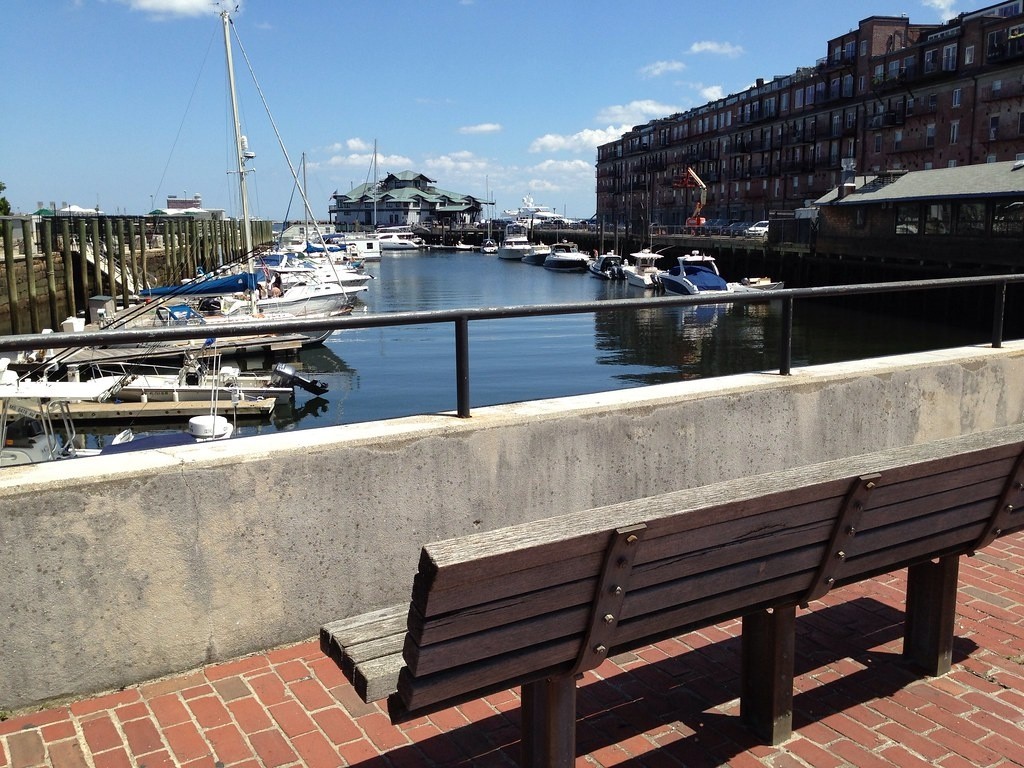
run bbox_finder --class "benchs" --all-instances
[316,421,1024,768]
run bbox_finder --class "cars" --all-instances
[537,216,770,242]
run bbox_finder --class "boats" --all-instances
[497,218,531,260]
[660,248,730,296]
[624,248,668,289]
[729,275,785,294]
[521,241,551,265]
[480,175,498,254]
[0,11,474,465]
[589,249,634,280]
[543,239,590,269]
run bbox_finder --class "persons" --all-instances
[272,272,280,288]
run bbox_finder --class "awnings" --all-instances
[813,159,1024,205]
